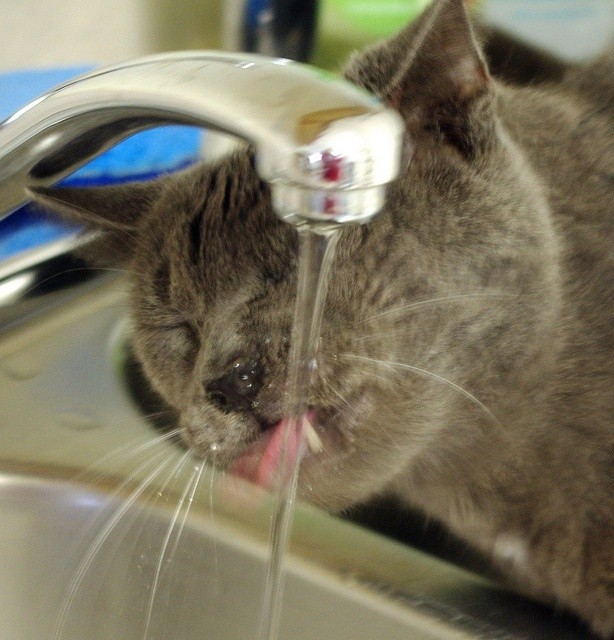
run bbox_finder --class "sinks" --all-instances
[0,458,614,637]
[0,235,570,602]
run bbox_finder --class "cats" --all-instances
[21,1,614,640]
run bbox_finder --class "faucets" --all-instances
[1,50,408,224]
